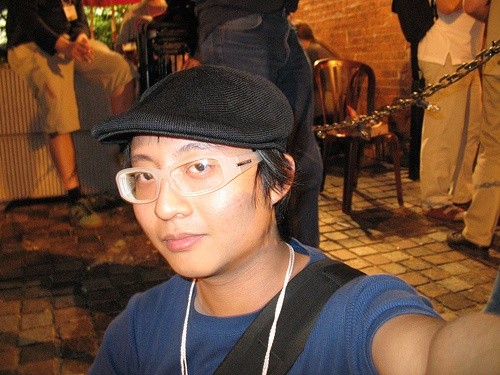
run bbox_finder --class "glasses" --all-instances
[115,150,264,202]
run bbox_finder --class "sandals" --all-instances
[421,203,468,222]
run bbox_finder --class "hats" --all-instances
[91,65,294,152]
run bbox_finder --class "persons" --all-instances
[5,0,137,228]
[117,0,168,72]
[444,0,500,258]
[193,0,321,250]
[79,63,500,375]
[418,0,489,226]
[293,22,347,118]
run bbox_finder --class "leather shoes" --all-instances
[447,230,490,258]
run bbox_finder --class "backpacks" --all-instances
[391,0,438,42]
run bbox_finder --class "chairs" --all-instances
[313,58,403,213]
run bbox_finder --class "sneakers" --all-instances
[67,194,103,229]
